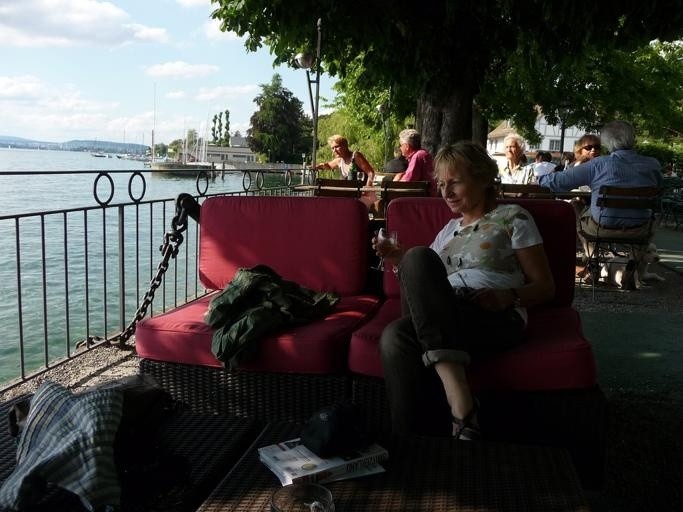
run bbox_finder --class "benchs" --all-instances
[308,166,589,260]
[659,174,682,229]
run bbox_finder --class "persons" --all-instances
[383,139,410,173]
[375,140,561,473]
[494,134,535,200]
[540,119,668,291]
[390,128,434,182]
[313,134,374,196]
[526,149,574,184]
[566,132,619,257]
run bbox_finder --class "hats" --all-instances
[301,404,366,457]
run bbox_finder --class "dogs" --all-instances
[579,242,667,290]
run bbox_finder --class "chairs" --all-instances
[578,184,660,304]
[346,195,609,455]
[134,197,381,431]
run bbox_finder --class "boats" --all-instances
[90,126,220,178]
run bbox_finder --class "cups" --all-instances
[356,171,365,182]
[269,481,336,512]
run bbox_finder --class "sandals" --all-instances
[450,394,484,441]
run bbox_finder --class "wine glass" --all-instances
[370,226,401,273]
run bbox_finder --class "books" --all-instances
[256,424,389,485]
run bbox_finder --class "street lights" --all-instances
[376,86,391,168]
[295,18,323,167]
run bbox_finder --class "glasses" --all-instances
[579,143,600,151]
